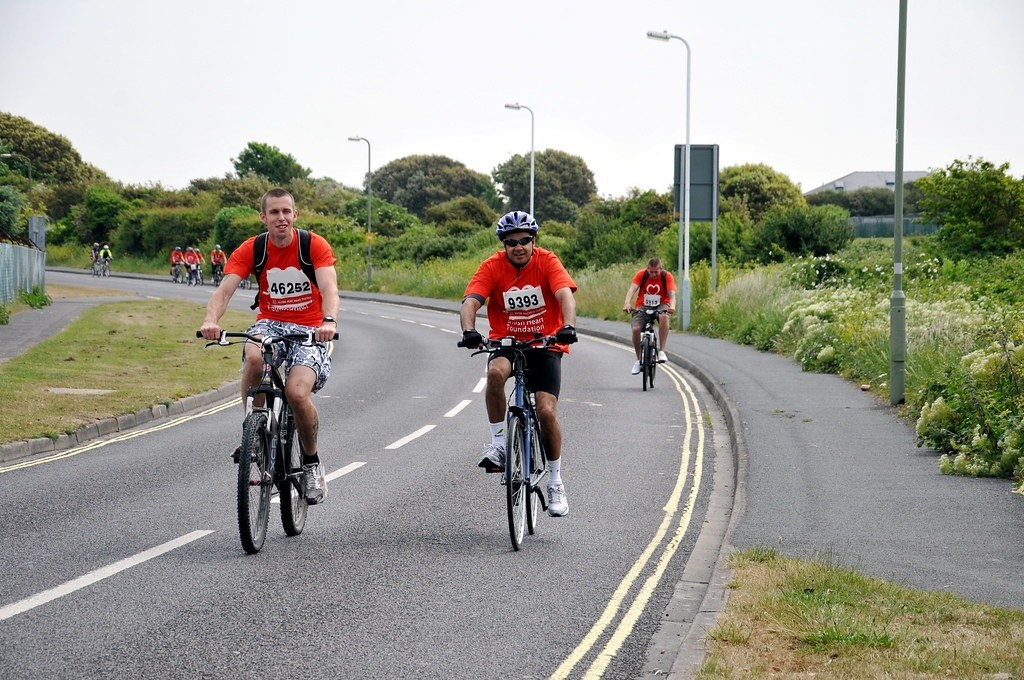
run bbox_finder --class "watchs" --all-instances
[322,315,337,328]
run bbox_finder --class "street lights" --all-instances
[1,154,32,214]
[504,103,534,220]
[348,137,372,282]
[647,30,691,331]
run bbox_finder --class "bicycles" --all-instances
[239,279,251,290]
[195,330,339,554]
[172,263,183,283]
[91,259,101,276]
[186,265,197,285]
[101,259,111,277]
[630,307,667,391]
[457,332,577,550]
[214,263,223,287]
[197,264,204,285]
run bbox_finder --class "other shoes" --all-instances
[230,446,256,457]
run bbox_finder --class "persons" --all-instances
[170,246,186,275]
[184,247,205,280]
[211,244,227,282]
[90,242,101,270]
[99,245,113,272]
[460,210,578,518]
[623,258,677,375]
[200,188,340,505]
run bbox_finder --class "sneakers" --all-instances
[632,360,642,375]
[658,350,667,361]
[547,484,569,517]
[302,452,327,505]
[478,443,505,468]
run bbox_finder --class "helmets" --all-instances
[215,244,220,249]
[186,247,193,252]
[104,245,109,249]
[496,211,539,242]
[503,236,534,246]
[94,242,99,246]
[175,247,181,251]
[195,248,200,253]
